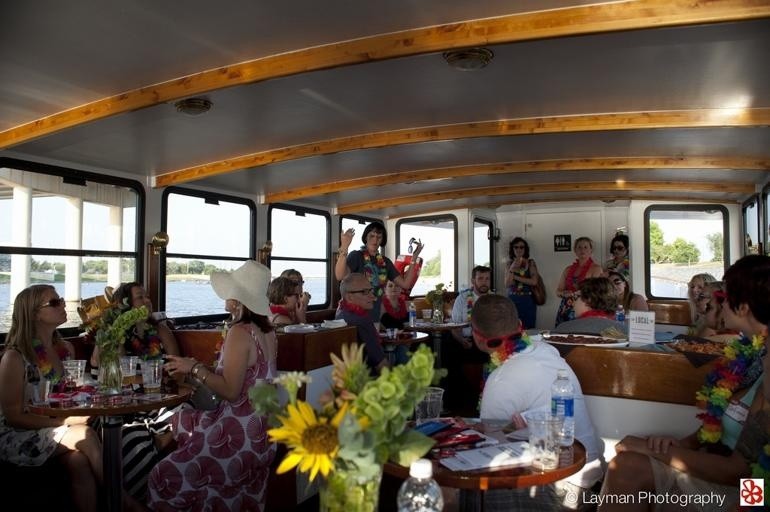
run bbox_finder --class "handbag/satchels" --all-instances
[531,275,545,305]
[191,378,222,411]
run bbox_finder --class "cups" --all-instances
[524,411,567,470]
[433,309,443,324]
[386,328,399,340]
[34,355,170,408]
[414,386,445,427]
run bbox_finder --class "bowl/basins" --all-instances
[421,308,432,319]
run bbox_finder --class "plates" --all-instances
[284,324,314,333]
[541,334,628,349]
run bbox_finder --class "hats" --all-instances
[211,260,274,316]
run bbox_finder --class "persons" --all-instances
[0,220,770,512]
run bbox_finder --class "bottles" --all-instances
[396,458,444,512]
[615,303,629,340]
[409,302,417,328]
[550,368,575,448]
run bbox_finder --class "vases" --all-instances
[432,301,445,324]
[94,347,122,398]
[317,459,381,512]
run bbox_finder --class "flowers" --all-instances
[76,297,154,379]
[240,339,442,487]
[426,280,458,305]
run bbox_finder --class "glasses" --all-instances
[408,238,416,253]
[613,245,624,250]
[714,290,728,304]
[474,324,522,349]
[348,288,374,295]
[515,246,524,249]
[614,279,622,285]
[40,297,64,309]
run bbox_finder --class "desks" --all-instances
[407,314,471,360]
[29,379,197,512]
[380,330,430,359]
[394,417,588,509]
